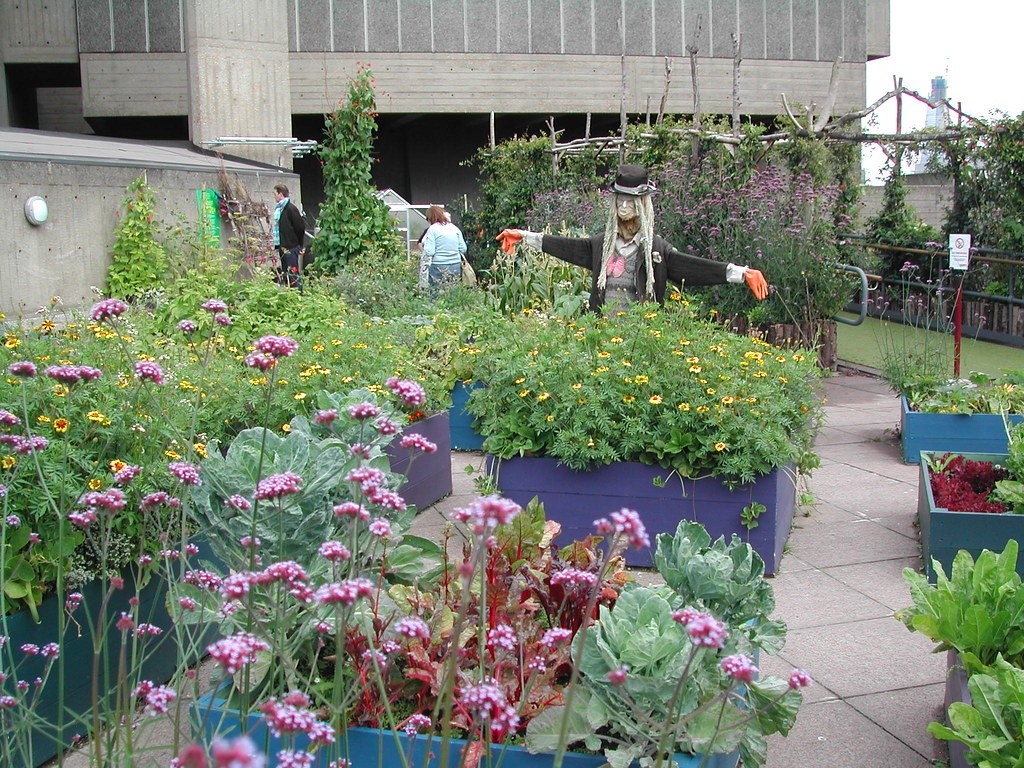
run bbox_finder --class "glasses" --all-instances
[426,217,428,221]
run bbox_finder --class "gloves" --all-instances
[743,268,769,301]
[496,228,527,253]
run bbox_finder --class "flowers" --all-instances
[0,276,1024,768]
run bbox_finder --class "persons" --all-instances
[494,164,770,319]
[272,184,305,287]
[417,207,467,293]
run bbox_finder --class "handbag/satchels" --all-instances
[459,252,476,289]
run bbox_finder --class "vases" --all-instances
[425,378,488,452]
[911,447,1024,584]
[483,448,796,579]
[375,407,452,515]
[0,535,230,768]
[896,390,1024,466]
[187,613,764,768]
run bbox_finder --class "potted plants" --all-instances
[893,540,1024,768]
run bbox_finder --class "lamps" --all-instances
[25,195,49,227]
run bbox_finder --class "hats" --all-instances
[607,165,657,196]
